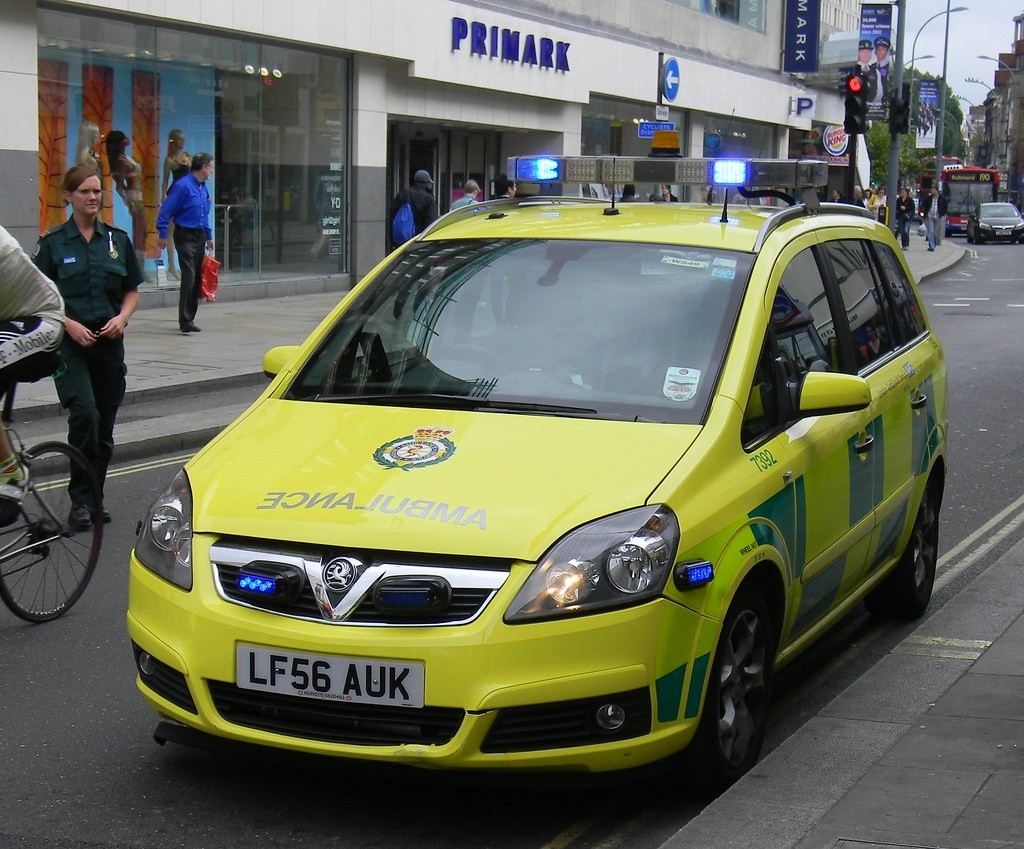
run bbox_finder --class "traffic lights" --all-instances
[843,72,873,135]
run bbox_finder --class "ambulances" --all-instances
[127,154,949,812]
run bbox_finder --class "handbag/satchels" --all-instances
[198,247,221,302]
[917,223,927,236]
[897,212,911,221]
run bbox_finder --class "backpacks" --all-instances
[392,189,427,245]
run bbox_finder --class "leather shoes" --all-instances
[89,505,112,521]
[68,502,92,526]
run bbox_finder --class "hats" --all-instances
[415,170,434,184]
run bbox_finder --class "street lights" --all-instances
[955,77,999,168]
[907,7,967,138]
[977,55,1016,200]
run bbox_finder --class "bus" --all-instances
[919,157,964,221]
[939,163,1000,238]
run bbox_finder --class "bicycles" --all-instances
[1,358,105,624]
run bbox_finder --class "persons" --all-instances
[807,325,900,395]
[923,187,947,252]
[447,179,483,213]
[895,189,916,251]
[619,183,638,204]
[853,186,887,222]
[392,170,437,322]
[76,120,102,176]
[107,131,152,285]
[495,174,518,201]
[162,129,192,278]
[829,190,843,203]
[0,221,64,527]
[699,186,718,201]
[857,40,882,105]
[156,153,214,334]
[871,37,891,100]
[31,165,144,529]
[660,184,677,201]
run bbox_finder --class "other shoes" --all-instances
[191,324,201,332]
[928,247,934,252]
[181,322,193,333]
[902,246,909,250]
[0,464,28,526]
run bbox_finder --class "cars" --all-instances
[966,203,1024,245]
